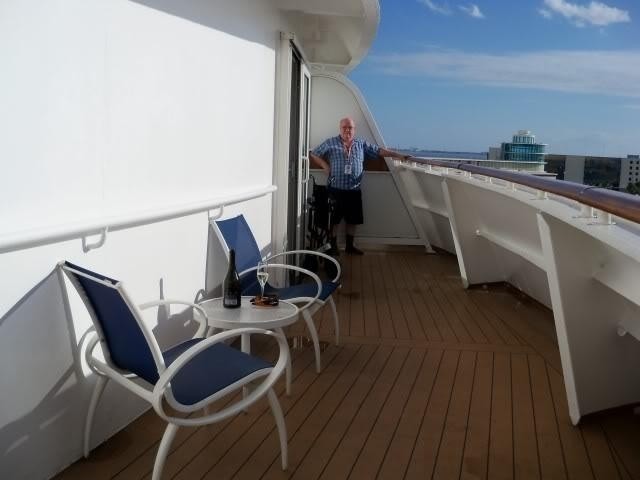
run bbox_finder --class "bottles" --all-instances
[222,249,242,308]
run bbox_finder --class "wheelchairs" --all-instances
[306,174,341,278]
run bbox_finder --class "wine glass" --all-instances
[257,261,269,301]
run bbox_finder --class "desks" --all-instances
[188,292,300,417]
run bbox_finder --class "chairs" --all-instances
[205,210,344,378]
[58,258,295,480]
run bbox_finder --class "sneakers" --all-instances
[322,247,340,256]
[344,246,363,256]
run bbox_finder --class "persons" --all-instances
[310,116,413,256]
